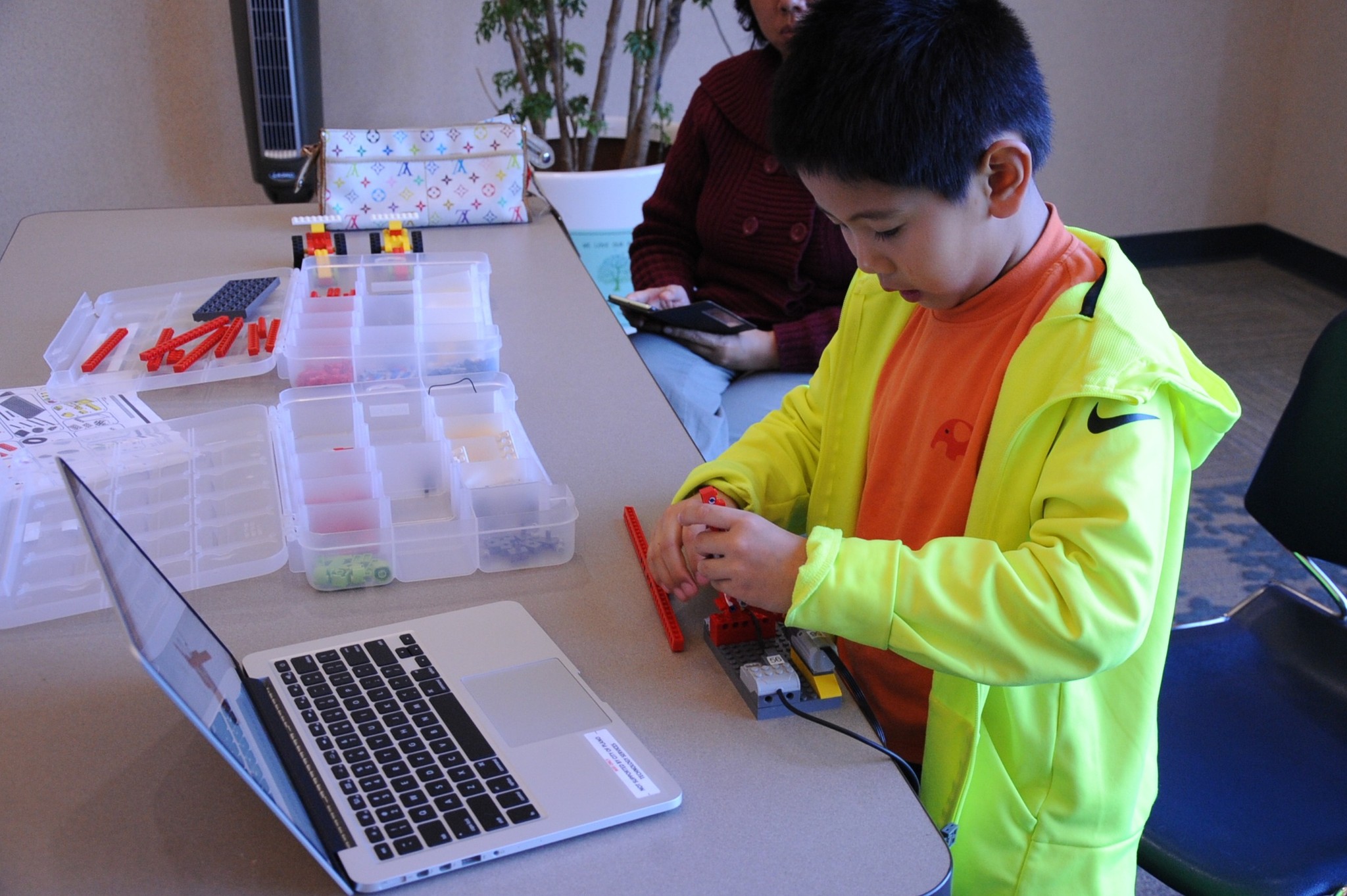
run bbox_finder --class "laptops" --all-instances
[55,456,682,896]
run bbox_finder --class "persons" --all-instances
[627,0,871,371]
[647,0,1243,896]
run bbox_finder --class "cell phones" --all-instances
[609,295,662,311]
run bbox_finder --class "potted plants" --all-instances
[472,0,756,230]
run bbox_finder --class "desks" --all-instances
[0,196,951,896]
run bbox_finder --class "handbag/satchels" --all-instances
[293,113,555,230]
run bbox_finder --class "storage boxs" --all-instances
[44,250,501,387]
[0,369,582,630]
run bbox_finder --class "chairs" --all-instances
[1136,312,1347,896]
